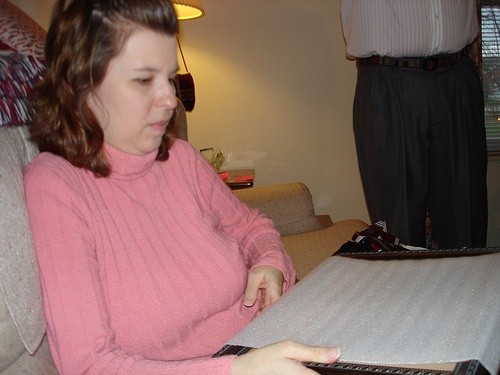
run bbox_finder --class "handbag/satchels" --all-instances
[173,71,197,113]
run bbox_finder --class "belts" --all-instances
[358,45,472,71]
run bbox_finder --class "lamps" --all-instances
[170,0,206,21]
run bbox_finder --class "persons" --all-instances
[341,0,487,249]
[23,0,341,375]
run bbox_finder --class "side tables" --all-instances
[220,168,255,189]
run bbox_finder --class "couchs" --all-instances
[0,0,370,375]
